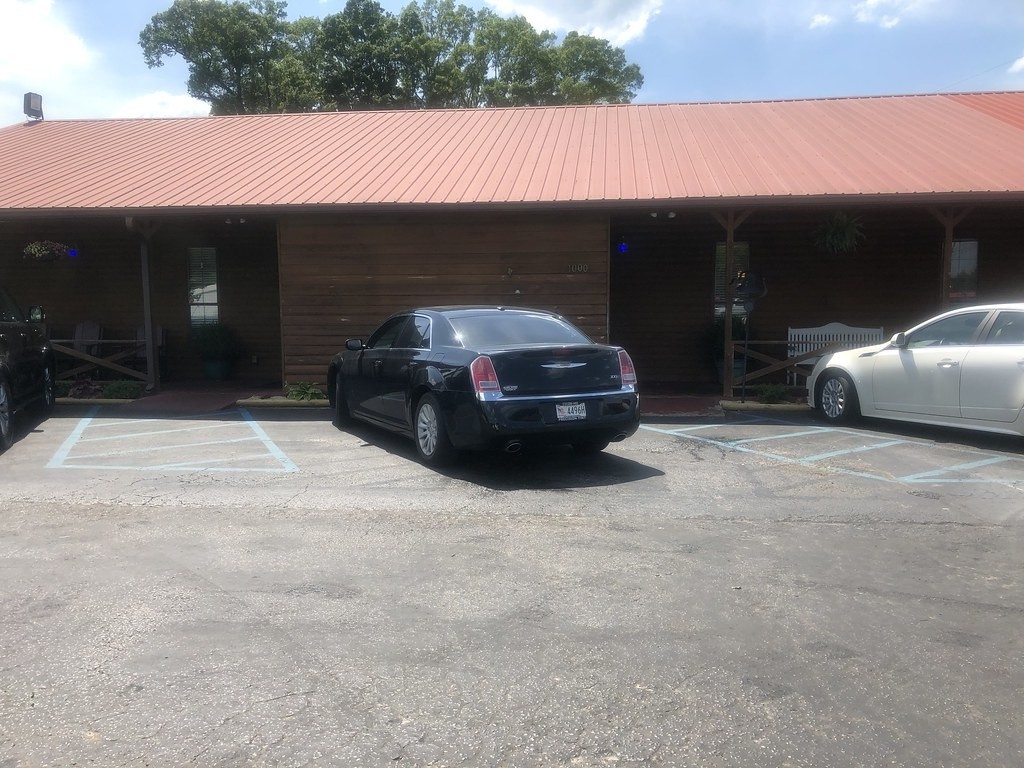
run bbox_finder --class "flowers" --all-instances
[24,240,79,260]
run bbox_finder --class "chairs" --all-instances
[121,321,169,379]
[57,319,104,379]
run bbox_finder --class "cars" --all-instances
[806,301,1024,439]
[0,281,59,451]
[325,305,641,463]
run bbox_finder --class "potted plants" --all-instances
[191,322,244,381]
